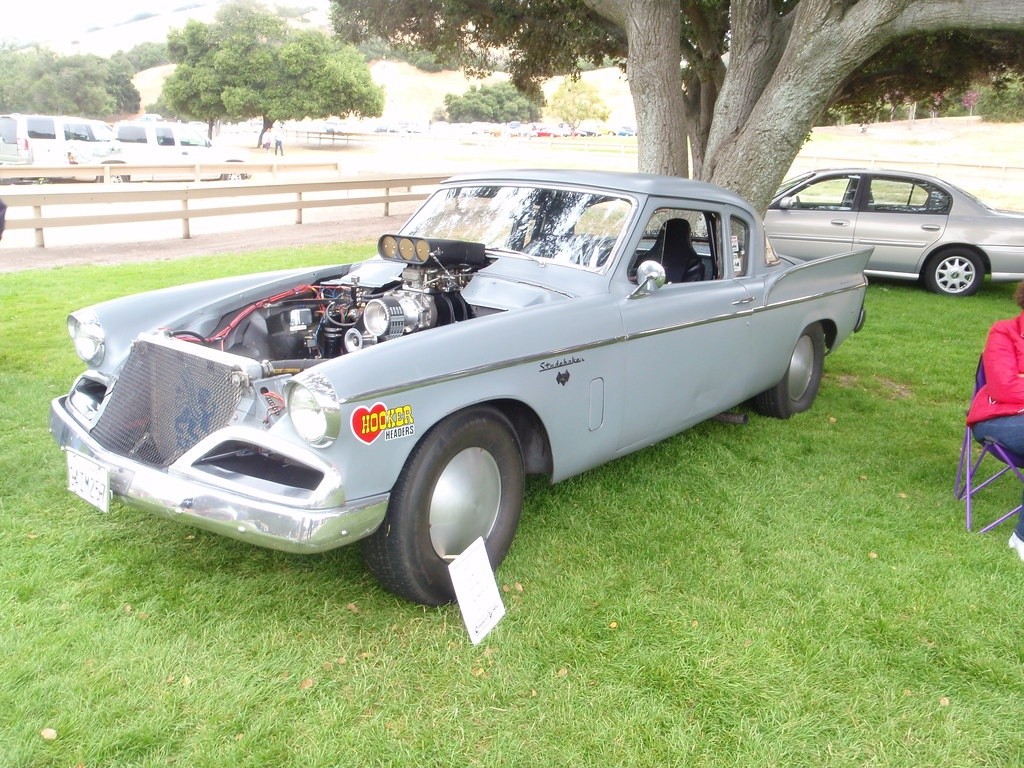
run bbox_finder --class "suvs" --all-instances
[0,114,262,184]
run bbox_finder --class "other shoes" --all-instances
[1008,531,1024,562]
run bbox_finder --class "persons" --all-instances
[263,121,285,157]
[966,280,1024,561]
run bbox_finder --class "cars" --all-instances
[763,165,1024,297]
[272,115,638,137]
[52,167,875,609]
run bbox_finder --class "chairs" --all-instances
[632,217,706,284]
[953,352,1024,536]
